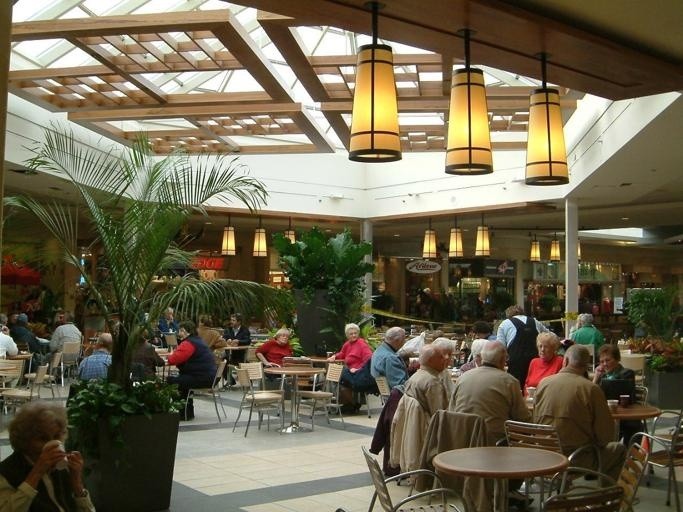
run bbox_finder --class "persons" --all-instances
[78,308,219,420]
[256,329,293,387]
[0,397,96,512]
[533,344,640,506]
[446,338,535,508]
[369,326,454,415]
[0,288,84,387]
[328,323,372,373]
[222,313,250,385]
[472,297,640,416]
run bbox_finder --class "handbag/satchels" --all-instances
[339,363,380,395]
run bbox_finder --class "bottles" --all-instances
[463,278,482,288]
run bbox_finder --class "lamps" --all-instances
[217,212,296,257]
[419,210,492,261]
[527,234,561,263]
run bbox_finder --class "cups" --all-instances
[447,366,461,377]
[607,400,619,413]
[169,328,172,333]
[43,439,68,471]
[526,387,537,398]
[409,358,420,365]
[620,394,630,408]
[58,314,64,323]
[326,352,333,358]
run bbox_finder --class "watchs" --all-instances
[73,488,89,498]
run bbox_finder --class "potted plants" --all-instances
[5,117,267,512]
[625,288,682,410]
[271,226,374,355]
